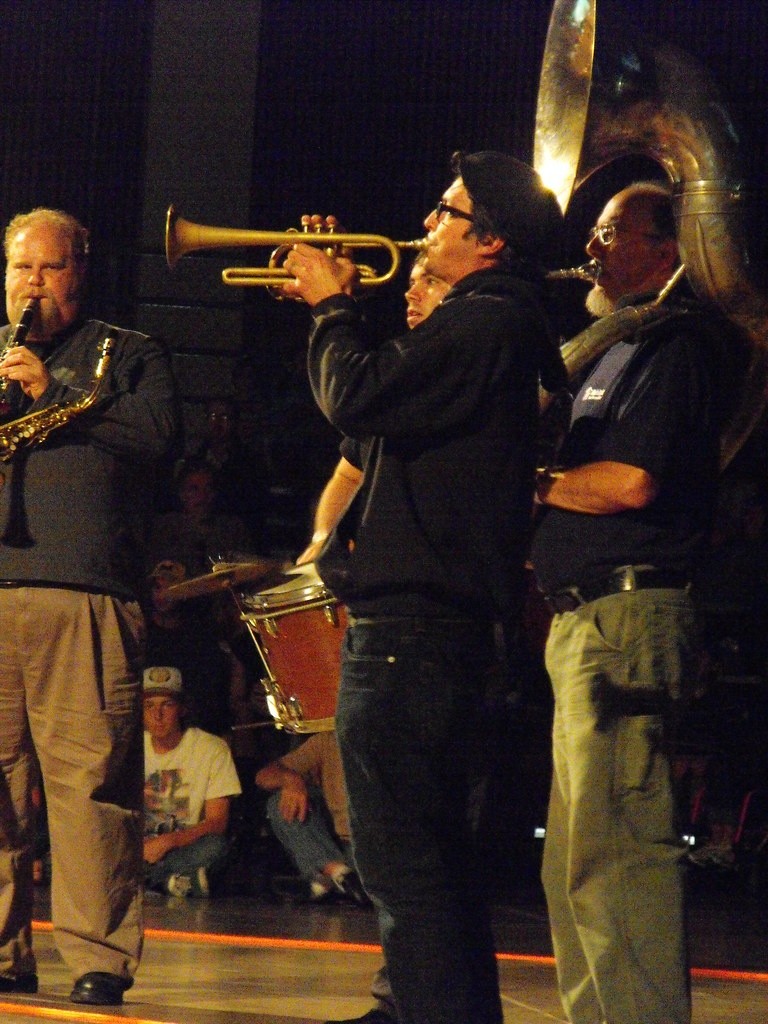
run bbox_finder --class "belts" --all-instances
[544,566,680,616]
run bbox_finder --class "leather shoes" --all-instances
[0,972,39,993]
[68,972,131,1006]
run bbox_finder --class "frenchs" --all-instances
[533,1,767,414]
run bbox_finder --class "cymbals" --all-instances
[154,558,292,604]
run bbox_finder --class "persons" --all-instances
[0,208,181,1003]
[280,150,566,1024]
[527,184,735,1024]
[21,249,768,901]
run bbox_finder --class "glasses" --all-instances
[433,200,490,233]
[588,224,663,246]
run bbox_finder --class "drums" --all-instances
[240,561,351,735]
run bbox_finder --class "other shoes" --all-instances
[165,866,211,901]
[276,867,373,911]
[319,1007,395,1024]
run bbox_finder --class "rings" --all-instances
[289,262,295,273]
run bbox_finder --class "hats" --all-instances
[144,560,188,586]
[445,148,556,254]
[141,665,189,703]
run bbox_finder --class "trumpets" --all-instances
[165,203,431,301]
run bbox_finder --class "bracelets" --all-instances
[533,466,554,505]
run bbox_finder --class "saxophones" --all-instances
[0,295,117,489]
